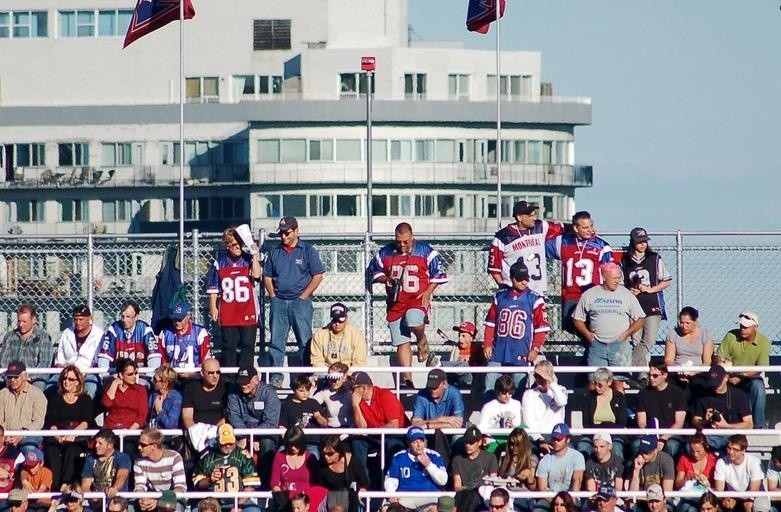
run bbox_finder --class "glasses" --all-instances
[590,381,607,388]
[123,371,139,377]
[488,503,506,509]
[206,370,221,375]
[278,230,294,237]
[648,371,663,379]
[724,446,740,452]
[738,314,758,326]
[350,386,361,392]
[6,375,20,378]
[121,314,136,319]
[320,450,337,457]
[137,442,156,448]
[396,239,411,245]
[499,390,512,395]
[62,376,78,382]
[553,502,568,507]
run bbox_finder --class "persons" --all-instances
[765,445,781,511]
[751,495,770,512]
[158,300,213,372]
[310,363,353,442]
[675,426,716,512]
[156,492,178,511]
[381,427,447,511]
[550,491,578,512]
[205,226,263,369]
[0,360,48,455]
[477,376,522,454]
[427,321,489,395]
[367,223,448,387]
[289,492,310,511]
[2,304,52,392]
[386,502,406,511]
[197,496,222,512]
[9,447,53,512]
[192,423,262,511]
[309,303,366,397]
[225,364,281,487]
[714,311,771,430]
[262,215,325,389]
[584,430,622,492]
[644,483,671,511]
[686,365,753,455]
[48,483,93,511]
[664,307,714,396]
[535,423,586,511]
[714,433,762,511]
[1,487,35,511]
[698,492,720,511]
[542,211,623,389]
[410,370,465,460]
[78,428,130,511]
[95,301,162,373]
[519,360,568,471]
[485,201,574,300]
[53,304,107,401]
[636,359,687,460]
[278,375,330,462]
[270,425,317,511]
[350,371,406,491]
[582,368,627,467]
[479,488,510,512]
[623,435,675,512]
[570,263,646,381]
[181,358,227,474]
[0,425,25,512]
[134,428,187,512]
[589,483,622,512]
[310,435,370,512]
[107,496,129,512]
[328,505,346,511]
[452,426,497,512]
[497,428,538,492]
[143,365,182,450]
[87,357,148,449]
[614,227,672,381]
[42,364,95,493]
[482,262,550,402]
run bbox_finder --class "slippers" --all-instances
[416,334,431,363]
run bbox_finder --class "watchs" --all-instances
[426,421,430,429]
[526,479,536,485]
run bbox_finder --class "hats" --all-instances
[456,424,483,443]
[425,368,447,389]
[645,483,664,501]
[21,449,44,467]
[550,423,571,440]
[407,426,425,442]
[734,311,758,327]
[169,302,191,321]
[217,423,236,445]
[591,485,616,501]
[509,262,530,282]
[73,304,91,316]
[635,433,657,452]
[329,302,348,323]
[2,359,26,376]
[452,321,478,338]
[235,365,258,384]
[630,227,651,242]
[706,365,726,389]
[276,216,298,232]
[593,430,613,445]
[512,200,539,217]
[343,371,373,387]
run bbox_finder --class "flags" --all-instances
[466,0,506,34]
[121,0,196,50]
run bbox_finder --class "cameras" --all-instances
[711,408,727,423]
[392,278,401,303]
